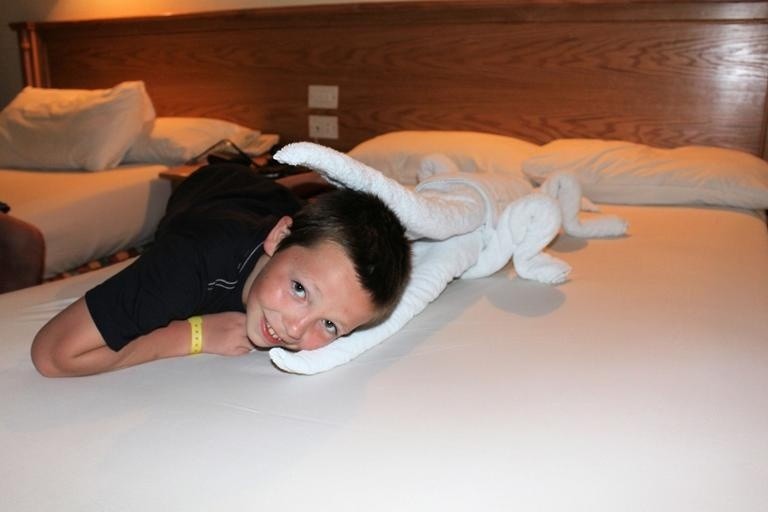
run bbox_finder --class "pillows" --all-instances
[341,129,545,197]
[0,80,281,172]
[522,133,766,208]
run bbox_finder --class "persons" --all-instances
[0,198,47,292]
[29,163,412,378]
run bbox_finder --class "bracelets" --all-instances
[188,315,202,355]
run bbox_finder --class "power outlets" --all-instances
[308,115,338,140]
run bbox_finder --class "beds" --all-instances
[0,165,768,512]
